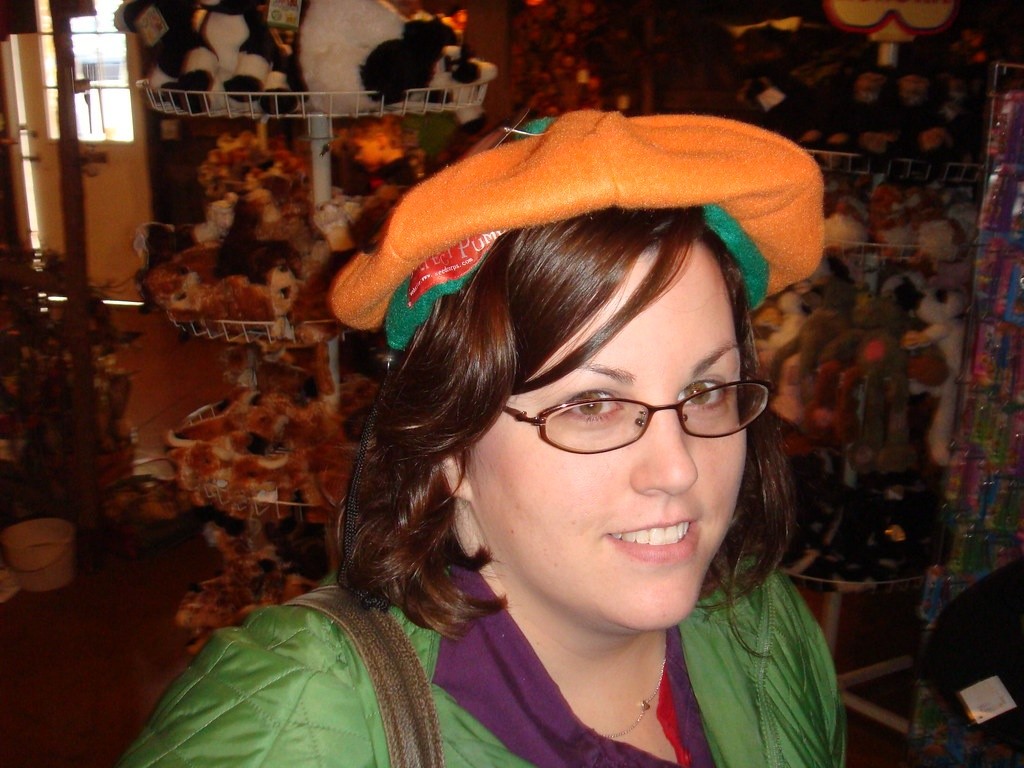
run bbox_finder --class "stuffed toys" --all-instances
[113,0,1024,591]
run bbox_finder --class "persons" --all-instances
[119,107,849,768]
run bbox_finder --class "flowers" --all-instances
[0,241,148,477]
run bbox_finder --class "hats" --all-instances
[330,110,825,350]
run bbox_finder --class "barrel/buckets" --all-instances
[0,518,76,591]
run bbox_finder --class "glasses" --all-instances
[502,367,774,454]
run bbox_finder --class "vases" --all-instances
[43,446,134,497]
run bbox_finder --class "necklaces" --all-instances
[592,653,670,740]
